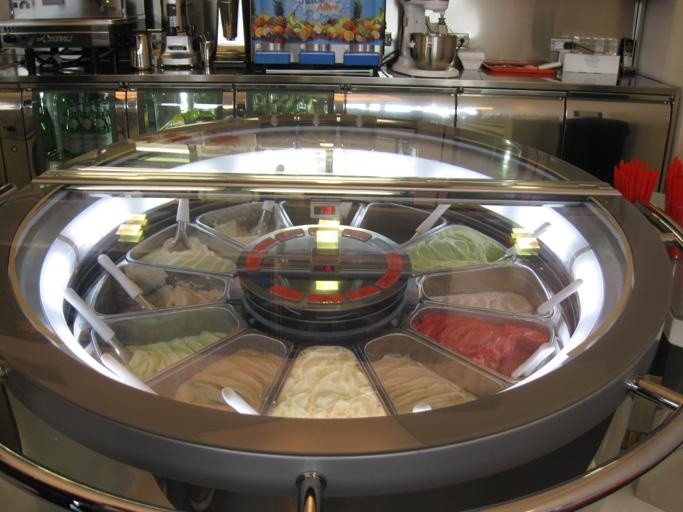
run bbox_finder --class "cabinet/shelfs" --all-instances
[456,84,566,161]
[344,83,457,127]
[32,83,126,176]
[566,83,681,194]
[126,84,235,139]
[235,84,345,119]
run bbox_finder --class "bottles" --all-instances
[37,92,113,157]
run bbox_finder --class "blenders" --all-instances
[390,0,461,78]
